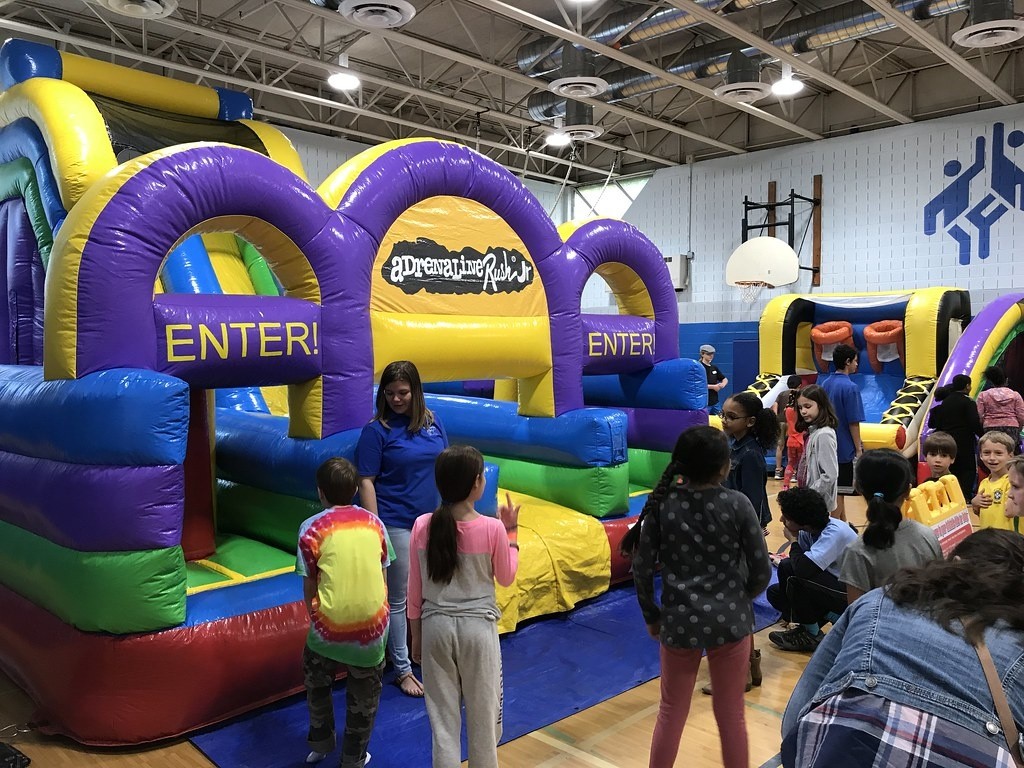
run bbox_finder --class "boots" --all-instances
[701,660,753,695]
[749,648,762,687]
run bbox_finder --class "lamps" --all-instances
[772,60,803,97]
[546,118,571,147]
[326,40,362,90]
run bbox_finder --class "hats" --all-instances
[699,344,715,354]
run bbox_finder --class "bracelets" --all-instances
[506,525,517,531]
[510,543,519,551]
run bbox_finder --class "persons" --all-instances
[1006,454,1024,517]
[720,393,782,528]
[698,345,729,414]
[976,366,1024,446]
[405,444,521,768]
[923,431,957,483]
[767,487,858,652]
[773,375,807,492]
[781,528,1024,768]
[355,361,450,697]
[295,457,392,768]
[621,426,772,768]
[971,430,1024,536]
[823,344,866,533]
[837,448,944,605]
[794,384,840,513]
[928,374,981,501]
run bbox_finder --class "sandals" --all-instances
[396,669,426,696]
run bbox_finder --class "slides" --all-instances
[1,76,714,757]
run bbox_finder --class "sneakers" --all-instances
[769,624,825,653]
[774,467,784,479]
[791,471,797,482]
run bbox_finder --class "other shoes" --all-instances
[307,748,326,762]
[848,522,859,534]
[363,751,371,766]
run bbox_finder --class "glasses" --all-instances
[718,410,749,421]
[383,387,411,400]
